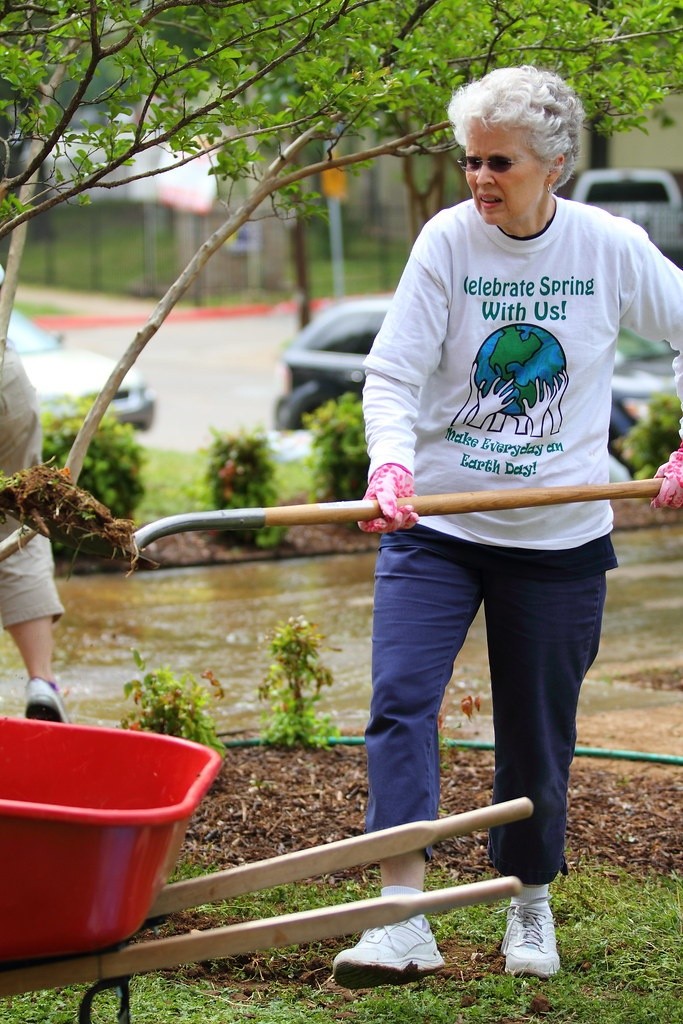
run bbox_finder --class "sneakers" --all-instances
[334,916,444,990]
[498,894,560,978]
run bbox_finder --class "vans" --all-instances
[1,305,159,435]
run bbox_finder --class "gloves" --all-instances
[357,463,420,534]
[651,442,683,510]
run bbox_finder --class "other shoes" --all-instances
[24,678,70,727]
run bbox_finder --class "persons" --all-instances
[0,264,69,723]
[332,66,683,989]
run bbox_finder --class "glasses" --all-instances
[456,156,531,172]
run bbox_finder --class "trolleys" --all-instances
[1,794,533,1024]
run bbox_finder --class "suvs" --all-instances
[568,168,683,268]
[276,294,673,459]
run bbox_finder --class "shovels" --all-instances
[0,477,664,571]
